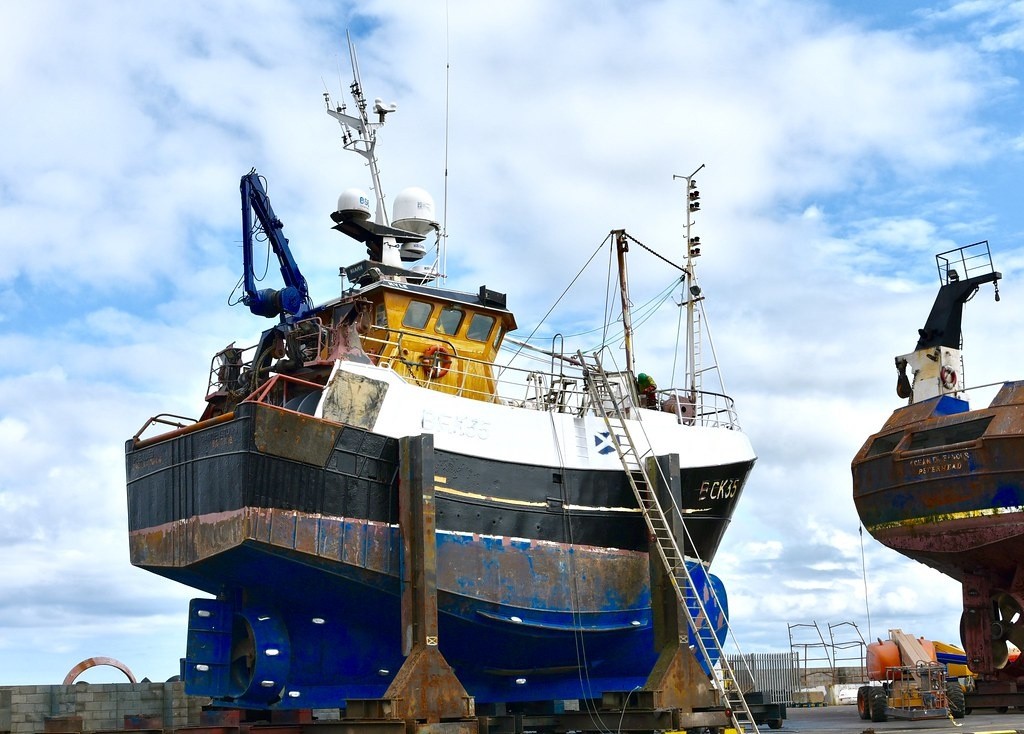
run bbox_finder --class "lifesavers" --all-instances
[423,345,451,378]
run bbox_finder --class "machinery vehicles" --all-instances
[858,628,965,722]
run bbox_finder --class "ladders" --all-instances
[577,349,759,734]
